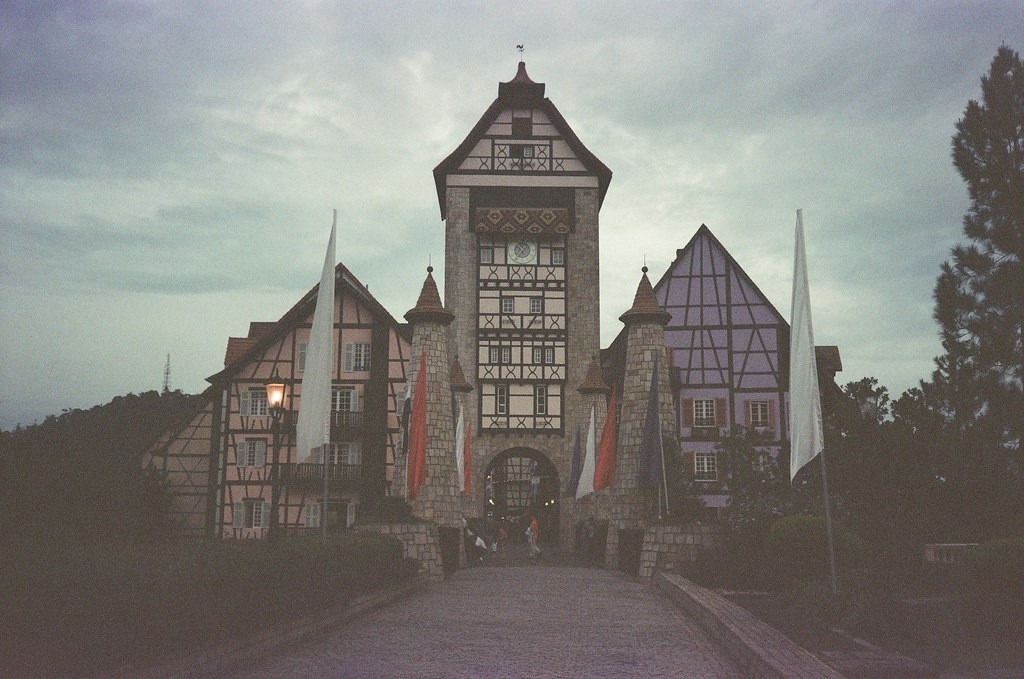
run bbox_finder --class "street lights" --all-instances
[267,365,291,534]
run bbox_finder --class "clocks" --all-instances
[507,237,536,264]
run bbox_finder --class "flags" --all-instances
[291,210,340,469]
[788,208,825,486]
[634,354,676,495]
[592,379,617,492]
[562,405,596,501]
[404,351,428,504]
[455,405,472,496]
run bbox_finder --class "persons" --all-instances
[494,521,510,563]
[524,512,544,559]
